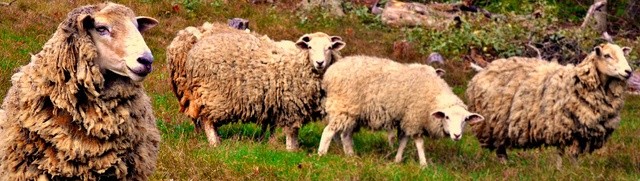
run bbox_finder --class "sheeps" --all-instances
[0,2,161,181]
[466,42,632,171]
[166,20,343,143]
[318,54,485,170]
[186,32,346,151]
